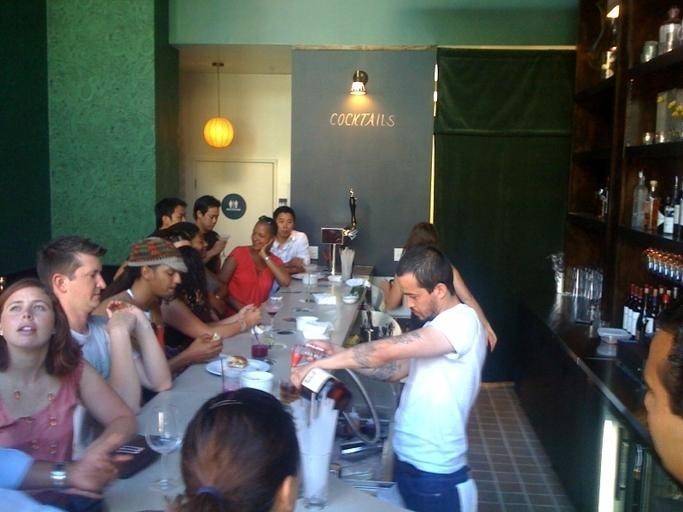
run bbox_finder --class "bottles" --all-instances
[622,283,678,339]
[658,8,682,55]
[300,367,353,413]
[632,169,682,238]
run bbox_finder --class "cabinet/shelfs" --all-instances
[514,0,682,512]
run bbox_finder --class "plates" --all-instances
[346,279,362,286]
[206,358,270,375]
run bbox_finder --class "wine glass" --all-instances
[145,403,183,493]
[640,246,683,283]
[262,303,283,325]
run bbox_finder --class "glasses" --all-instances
[260,216,273,225]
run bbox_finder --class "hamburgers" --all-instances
[226,356,247,369]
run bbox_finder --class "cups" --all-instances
[279,378,300,402]
[305,322,330,339]
[569,264,602,301]
[296,316,319,330]
[242,360,274,393]
[250,333,268,358]
[220,358,242,393]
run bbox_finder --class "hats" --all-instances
[128,236,188,272]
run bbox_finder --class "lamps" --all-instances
[203,62,234,149]
[350,69,369,95]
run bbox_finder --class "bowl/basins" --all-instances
[342,296,359,303]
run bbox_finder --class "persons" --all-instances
[92,236,223,409]
[113,193,312,322]
[290,239,488,511]
[0,277,138,463]
[102,242,262,374]
[0,445,134,512]
[36,234,172,449]
[375,222,498,353]
[160,387,302,512]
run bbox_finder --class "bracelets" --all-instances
[236,317,247,332]
[50,462,68,489]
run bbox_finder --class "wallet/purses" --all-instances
[109,435,162,479]
